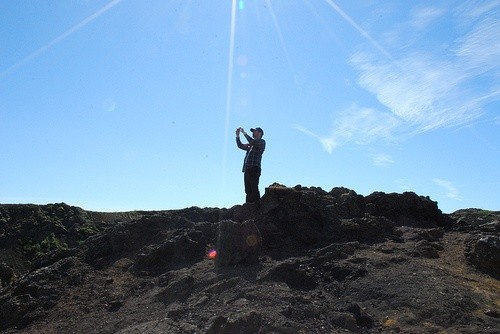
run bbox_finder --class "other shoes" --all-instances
[243,202,255,207]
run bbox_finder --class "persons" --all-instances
[235,127,266,202]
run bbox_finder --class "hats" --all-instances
[250,128,264,135]
